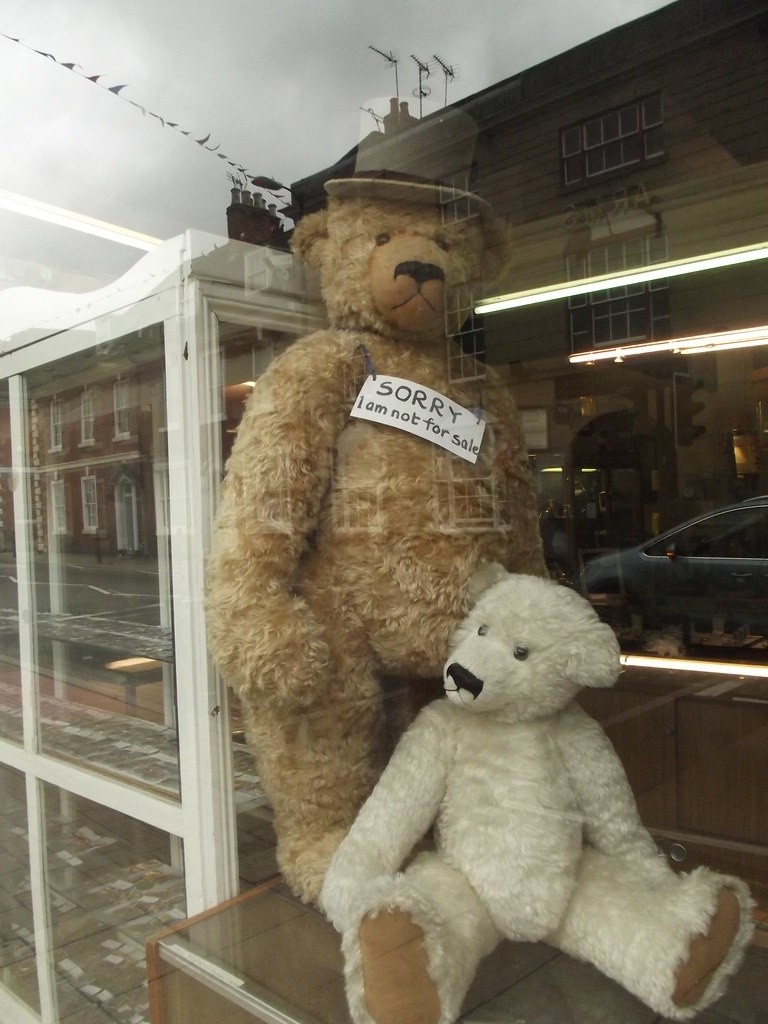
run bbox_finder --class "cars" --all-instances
[578,494,767,636]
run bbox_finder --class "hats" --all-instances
[322,97,496,223]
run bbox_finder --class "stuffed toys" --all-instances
[319,561,755,1024]
[203,135,551,912]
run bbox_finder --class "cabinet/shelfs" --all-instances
[0,228,346,1024]
